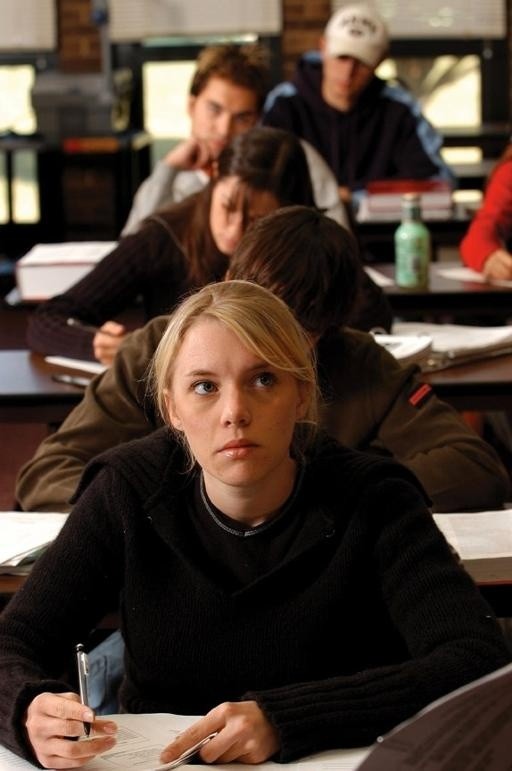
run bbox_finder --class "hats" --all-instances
[323,6,388,68]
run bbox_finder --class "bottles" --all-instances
[394,192,432,290]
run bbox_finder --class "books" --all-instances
[432,508,511,584]
[367,333,433,371]
[12,240,126,305]
[395,317,512,372]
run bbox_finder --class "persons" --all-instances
[254,5,458,219]
[0,276,511,771]
[9,203,511,515]
[454,143,511,288]
[114,38,355,238]
[20,122,395,368]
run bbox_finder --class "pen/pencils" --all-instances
[77,643,90,739]
[67,319,97,334]
[497,224,506,248]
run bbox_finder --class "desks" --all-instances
[0,128,512,771]
